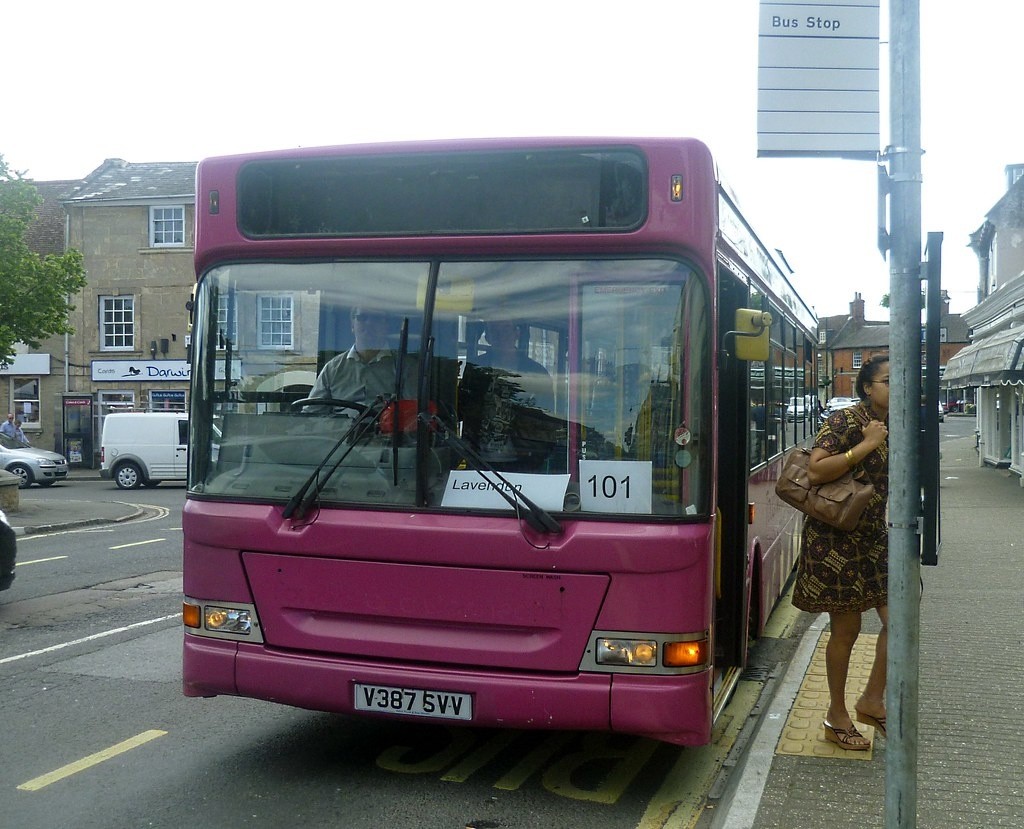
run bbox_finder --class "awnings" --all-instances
[941,325,1024,387]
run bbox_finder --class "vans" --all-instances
[98,405,221,490]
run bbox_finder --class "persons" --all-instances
[299,301,422,435]
[792,352,891,751]
[14,420,30,447]
[0,413,17,438]
[458,311,553,474]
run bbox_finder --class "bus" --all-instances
[182,136,827,750]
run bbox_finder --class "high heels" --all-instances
[823,720,871,749]
[857,707,886,739]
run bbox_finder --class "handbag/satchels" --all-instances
[775,410,874,531]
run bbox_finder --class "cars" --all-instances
[938,400,946,422]
[786,394,817,423]
[0,430,69,490]
[818,396,860,428]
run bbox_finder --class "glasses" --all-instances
[866,378,890,387]
[353,312,386,324]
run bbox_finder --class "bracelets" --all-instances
[845,449,858,469]
